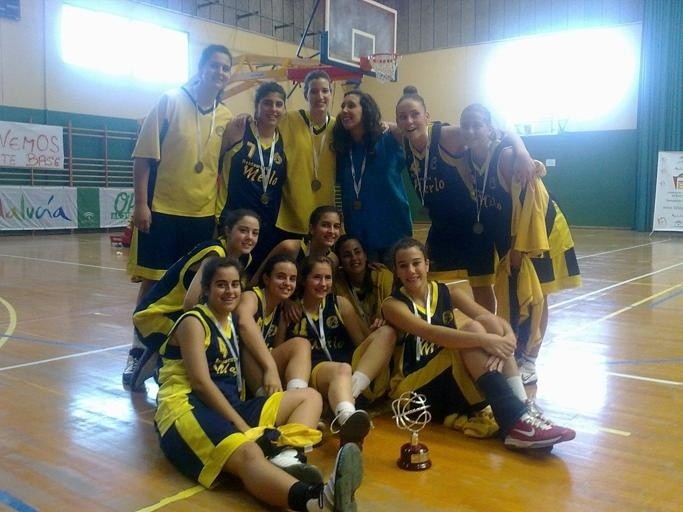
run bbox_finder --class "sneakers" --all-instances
[330,407,375,454]
[504,413,576,450]
[305,442,364,512]
[516,357,537,385]
[264,445,324,487]
[123,348,160,392]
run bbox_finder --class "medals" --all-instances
[351,199,362,210]
[311,180,322,192]
[417,204,429,216]
[473,222,484,233]
[194,161,205,174]
[261,194,270,204]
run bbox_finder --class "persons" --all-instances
[458,104,580,385]
[130,207,394,511]
[122,44,232,385]
[384,237,576,450]
[333,90,414,263]
[218,81,287,255]
[384,86,534,314]
[234,69,336,242]
